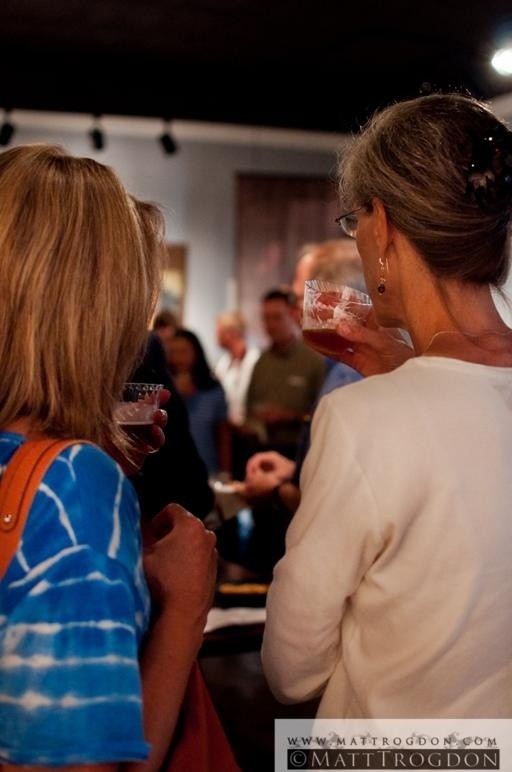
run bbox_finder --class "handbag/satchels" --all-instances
[0,653,240,772]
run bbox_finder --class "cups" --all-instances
[114,382,165,446]
[300,278,374,360]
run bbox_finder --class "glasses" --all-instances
[334,209,366,238]
